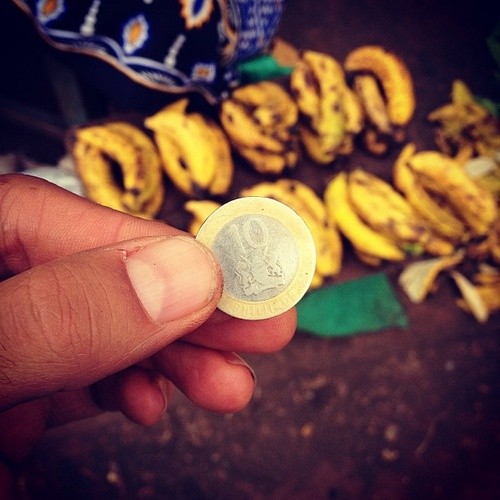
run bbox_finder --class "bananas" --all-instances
[71,46,499,323]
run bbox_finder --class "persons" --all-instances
[0,167,300,458]
